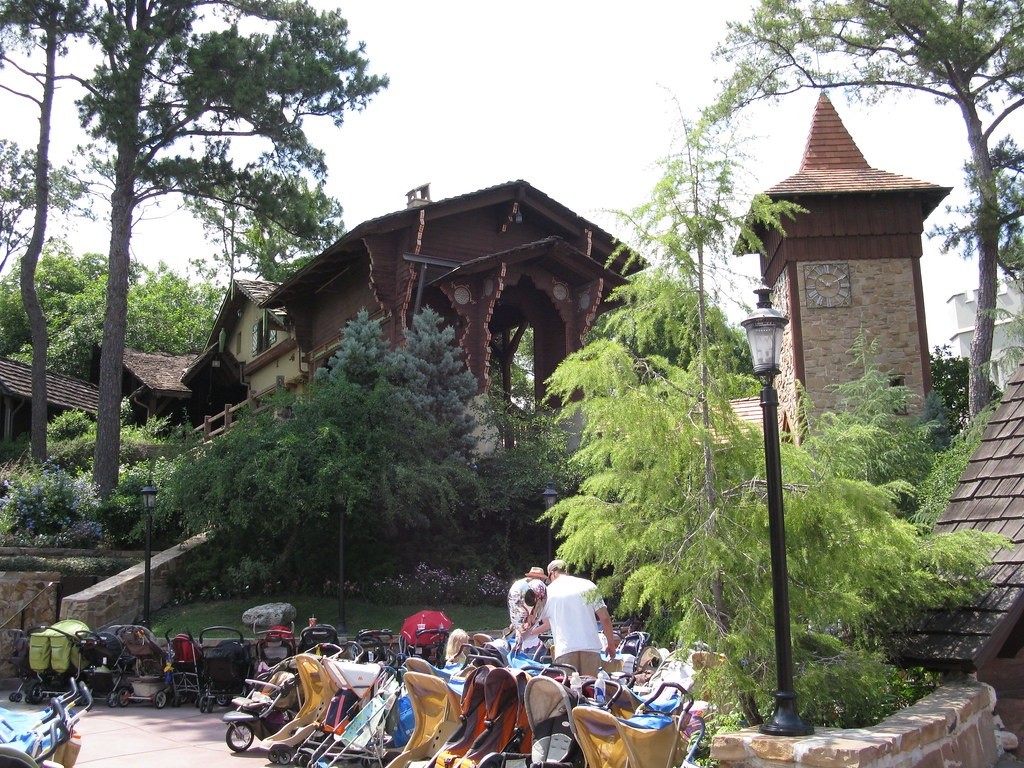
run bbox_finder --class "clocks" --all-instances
[803,264,852,308]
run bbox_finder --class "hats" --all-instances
[547,560,566,578]
[525,567,547,579]
[528,579,546,598]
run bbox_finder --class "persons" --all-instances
[507,560,616,678]
[445,628,470,663]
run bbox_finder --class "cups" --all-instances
[309,618,317,627]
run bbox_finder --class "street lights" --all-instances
[143,477,158,628]
[738,284,816,737]
[544,482,557,568]
[335,488,352,618]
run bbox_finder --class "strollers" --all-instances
[1,609,719,767]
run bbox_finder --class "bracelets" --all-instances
[530,630,533,635]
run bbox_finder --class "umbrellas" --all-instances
[400,609,454,646]
[164,644,174,683]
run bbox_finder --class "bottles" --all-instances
[570,672,582,693]
[598,667,610,680]
[594,673,605,702]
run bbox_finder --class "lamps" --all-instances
[237,309,243,318]
[515,207,522,222]
